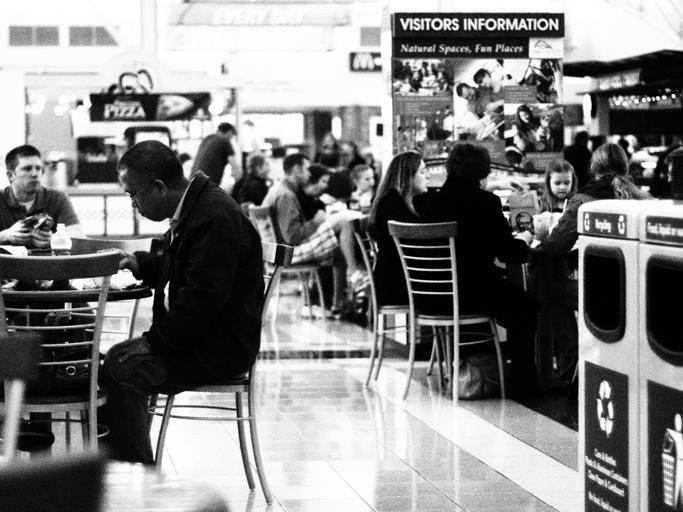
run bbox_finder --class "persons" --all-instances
[402,62,449,93]
[456,67,512,118]
[0,144,101,341]
[183,122,243,192]
[525,60,555,96]
[233,103,683,405]
[95,138,266,468]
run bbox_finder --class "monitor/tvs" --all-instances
[132,127,171,148]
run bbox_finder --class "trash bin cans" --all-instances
[575,200,683,512]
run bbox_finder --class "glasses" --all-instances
[125,187,152,199]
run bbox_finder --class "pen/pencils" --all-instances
[31,248,52,253]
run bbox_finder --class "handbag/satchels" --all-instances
[444,353,500,400]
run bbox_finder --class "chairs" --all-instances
[247,203,326,322]
[350,219,510,410]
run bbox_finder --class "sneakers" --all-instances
[346,268,370,291]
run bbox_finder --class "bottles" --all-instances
[49,223,73,290]
